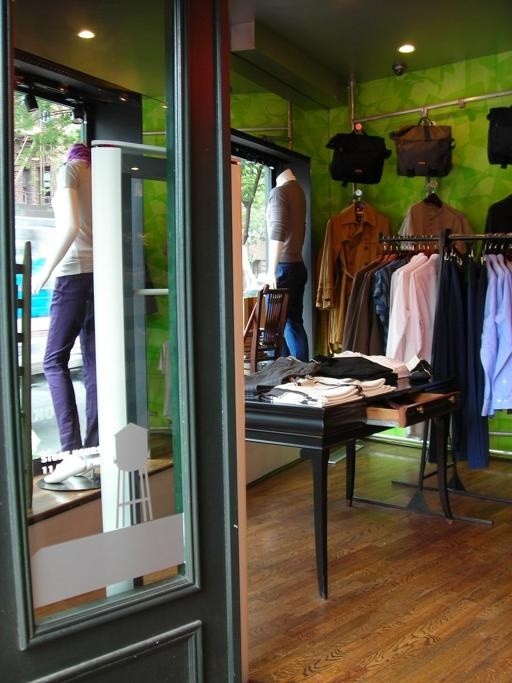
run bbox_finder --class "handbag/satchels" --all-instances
[389,117,456,177]
[325,128,391,188]
[489,106,512,165]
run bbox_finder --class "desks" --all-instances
[245,388,467,599]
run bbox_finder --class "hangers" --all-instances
[421,183,442,208]
[374,233,511,269]
[351,190,366,212]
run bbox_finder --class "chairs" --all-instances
[243,284,291,374]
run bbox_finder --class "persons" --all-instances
[28,140,101,491]
[265,164,311,364]
